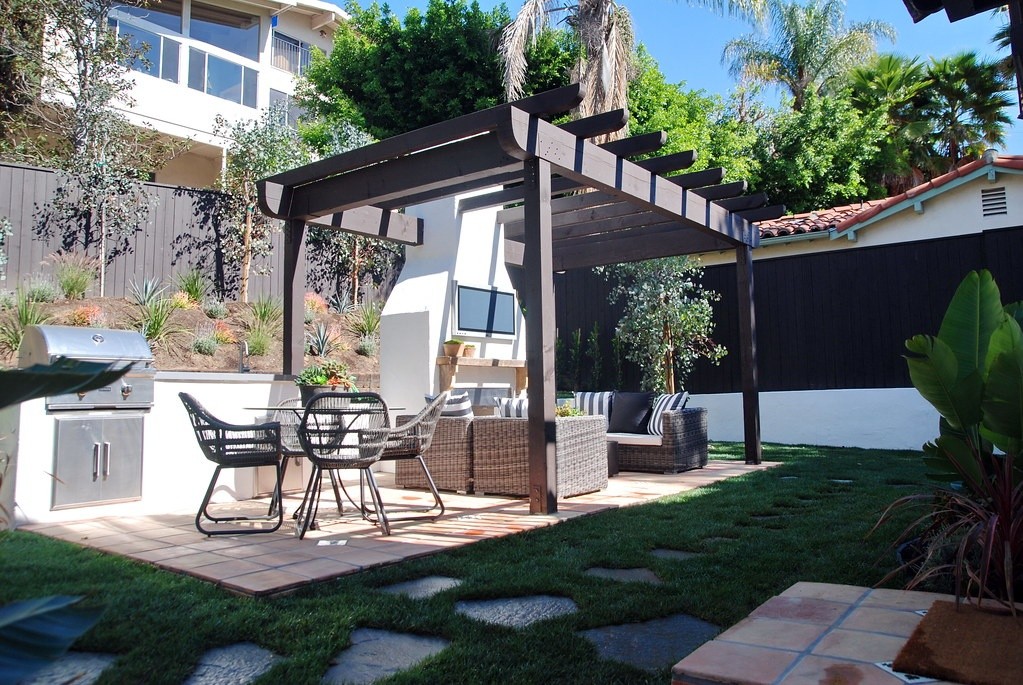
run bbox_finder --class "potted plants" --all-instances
[443,338,464,356]
[463,345,476,357]
[293,359,361,409]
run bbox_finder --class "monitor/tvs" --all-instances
[452,281,517,341]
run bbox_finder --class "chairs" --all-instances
[358,391,448,522]
[178,392,283,537]
[267,397,345,521]
[296,392,391,540]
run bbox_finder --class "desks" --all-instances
[243,407,406,530]
[607,441,619,478]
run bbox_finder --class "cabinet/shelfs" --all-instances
[50,413,145,512]
[436,356,528,394]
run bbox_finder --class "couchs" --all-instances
[471,413,607,499]
[395,414,474,496]
[605,407,709,476]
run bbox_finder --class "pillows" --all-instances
[492,397,528,417]
[571,391,613,433]
[606,391,657,434]
[424,390,475,416]
[646,391,691,436]
